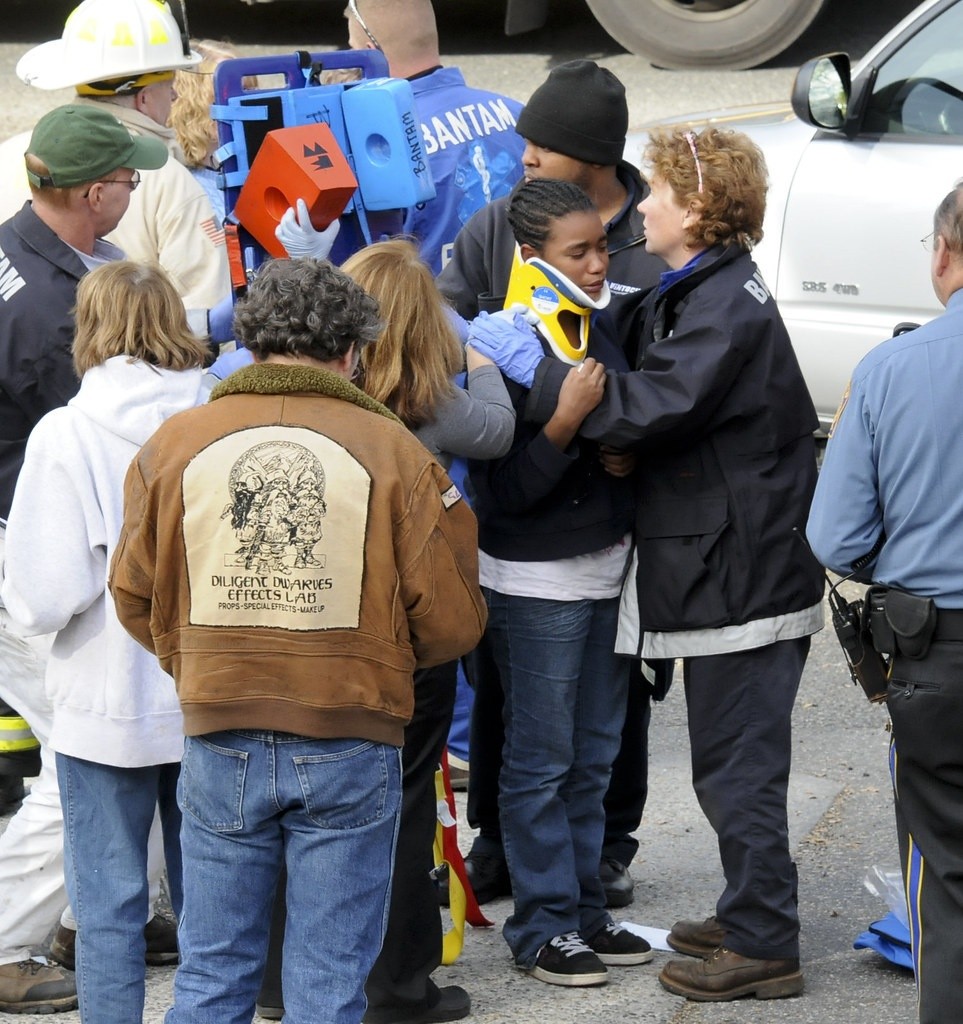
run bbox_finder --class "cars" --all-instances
[625,0,963,438]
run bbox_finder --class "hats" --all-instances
[514,58,628,165]
[24,104,168,189]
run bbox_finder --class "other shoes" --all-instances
[363,984,470,1024]
[599,850,635,908]
[436,832,511,906]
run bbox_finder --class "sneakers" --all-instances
[49,913,180,968]
[525,932,608,985]
[659,946,804,1003]
[589,921,654,964]
[0,957,78,1013]
[666,915,725,956]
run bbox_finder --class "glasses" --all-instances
[920,231,936,252]
[681,130,704,194]
[343,0,385,54]
[82,170,141,199]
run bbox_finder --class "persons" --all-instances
[802,178,962,1024]
[467,129,831,1006]
[0,0,660,1023]
[103,252,487,1024]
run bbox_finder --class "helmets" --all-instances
[15,0,204,91]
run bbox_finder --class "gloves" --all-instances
[465,305,545,389]
[273,196,340,265]
